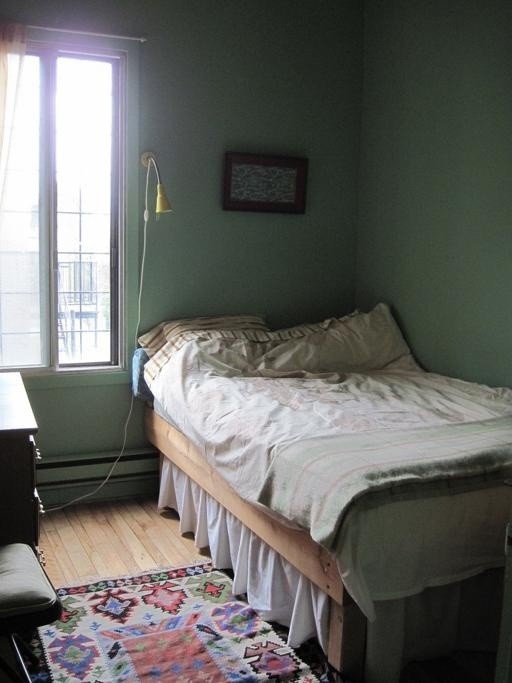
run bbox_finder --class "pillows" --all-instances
[139,316,271,351]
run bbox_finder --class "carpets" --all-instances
[14,564,344,681]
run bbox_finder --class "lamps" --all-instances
[141,151,173,214]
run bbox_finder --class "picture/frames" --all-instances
[222,152,309,215]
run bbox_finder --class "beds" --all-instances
[137,316,511,681]
